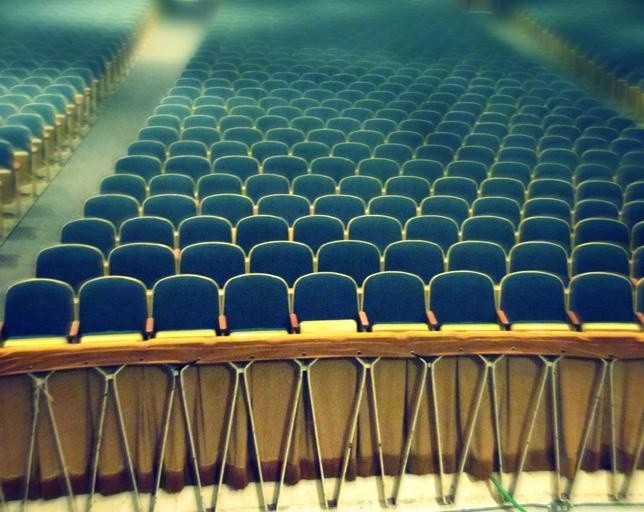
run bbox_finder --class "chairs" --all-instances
[0,0,643,512]
